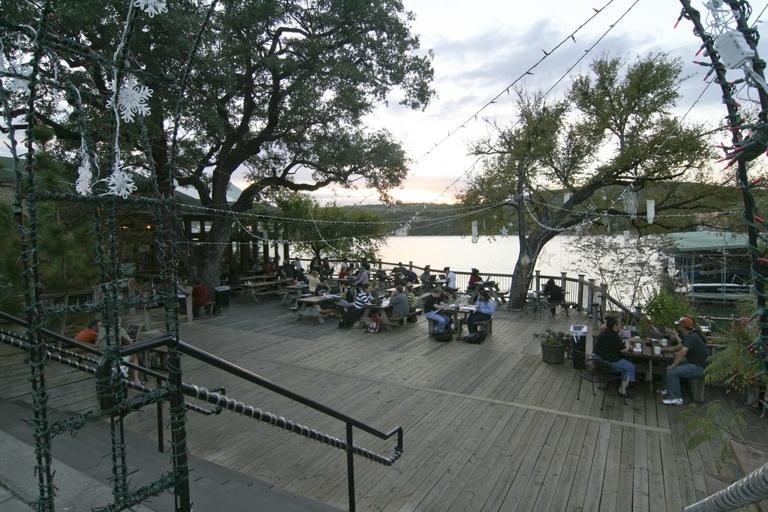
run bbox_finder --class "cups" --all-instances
[654,347,660,355]
[661,339,668,346]
[635,343,642,351]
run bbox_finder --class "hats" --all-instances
[674,317,692,330]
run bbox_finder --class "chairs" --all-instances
[574,349,627,411]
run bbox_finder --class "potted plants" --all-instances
[533,329,572,364]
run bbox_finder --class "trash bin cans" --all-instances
[215,286,231,310]
[570,324,587,371]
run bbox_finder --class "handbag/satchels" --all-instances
[463,332,480,344]
[436,333,452,342]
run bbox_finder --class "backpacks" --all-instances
[366,311,382,333]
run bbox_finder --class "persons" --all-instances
[193,279,210,321]
[72,320,100,343]
[591,311,710,405]
[266,253,496,335]
[96,315,148,386]
[543,278,563,317]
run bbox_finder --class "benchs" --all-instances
[176,301,215,319]
[231,275,509,340]
[688,376,704,401]
[523,302,575,320]
[40,288,95,311]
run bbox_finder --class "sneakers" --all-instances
[662,398,683,406]
[655,388,668,396]
